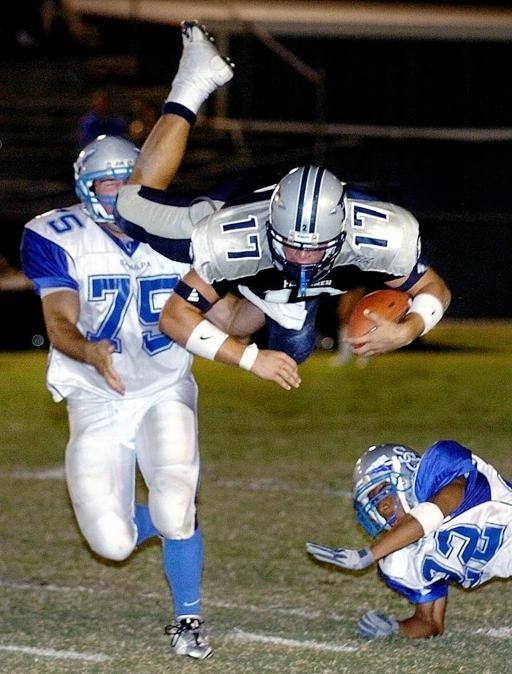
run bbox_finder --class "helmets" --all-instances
[266,165,348,271]
[353,443,425,539]
[74,133,140,224]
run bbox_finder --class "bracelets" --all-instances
[405,293,444,337]
[186,319,230,362]
[239,342,260,370]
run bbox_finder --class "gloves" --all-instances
[357,610,399,639]
[304,540,375,574]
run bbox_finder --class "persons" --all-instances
[112,19,452,390]
[18,134,267,661]
[306,438,512,640]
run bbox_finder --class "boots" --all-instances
[165,19,235,114]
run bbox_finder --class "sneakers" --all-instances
[174,616,212,661]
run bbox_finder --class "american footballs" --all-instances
[348,290,414,352]
